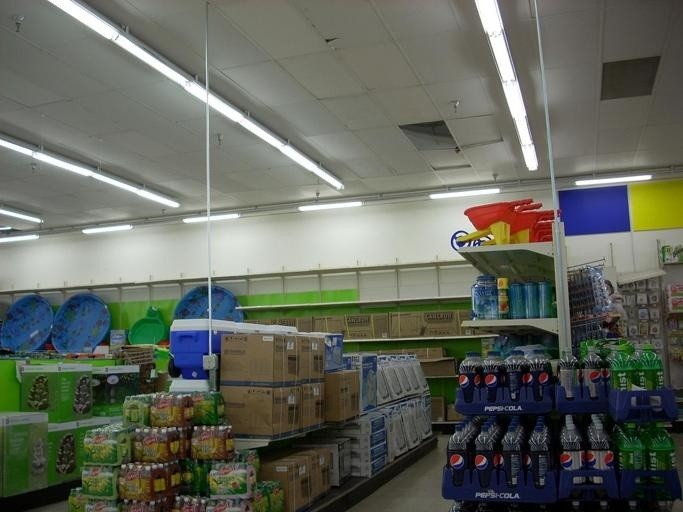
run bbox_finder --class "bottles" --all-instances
[68,461,285,511]
[123,390,228,427]
[454,343,667,404]
[618,418,678,488]
[470,273,553,320]
[447,418,550,489]
[559,415,616,486]
[88,425,261,462]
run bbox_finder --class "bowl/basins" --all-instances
[0,295,53,353]
[173,286,244,322]
[51,292,112,354]
[128,318,165,344]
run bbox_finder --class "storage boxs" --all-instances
[379,349,402,355]
[431,396,445,422]
[219,333,302,388]
[312,315,345,334]
[271,325,295,337]
[428,346,444,359]
[302,382,326,434]
[301,332,344,375]
[324,370,359,423]
[219,385,302,440]
[243,319,271,325]
[49,421,79,489]
[446,403,464,421]
[94,363,141,417]
[0,412,49,498]
[350,429,386,450]
[343,352,377,414]
[20,363,94,423]
[272,318,295,327]
[0,358,26,414]
[293,437,352,488]
[351,443,387,462]
[327,415,386,435]
[349,455,387,479]
[422,310,457,337]
[418,357,457,376]
[78,416,110,480]
[402,347,426,359]
[296,318,313,332]
[236,322,266,336]
[388,311,422,337]
[457,309,472,336]
[299,335,325,385]
[169,317,237,379]
[278,446,332,499]
[344,313,389,339]
[257,455,313,512]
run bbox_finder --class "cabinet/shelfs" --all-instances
[456,222,573,365]
[168,356,438,512]
